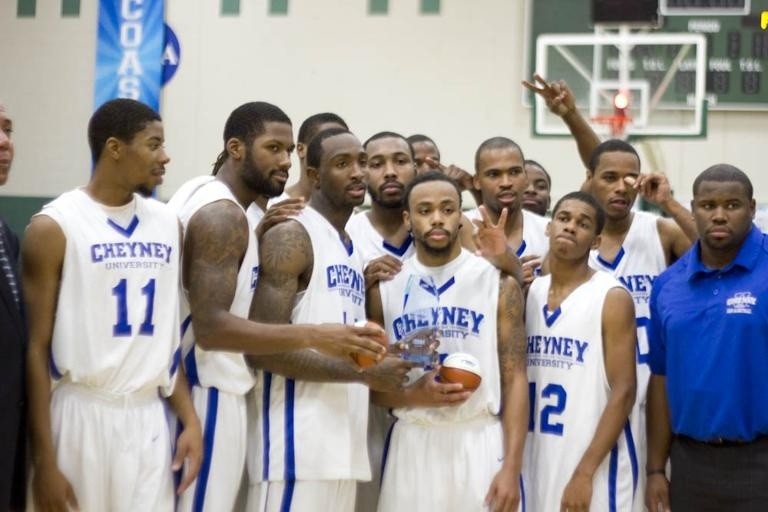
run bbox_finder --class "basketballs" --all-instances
[349,319,392,370]
[441,351,483,396]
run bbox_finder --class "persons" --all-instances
[19,99,205,511]
[509,74,609,222]
[645,162,766,510]
[1,100,29,512]
